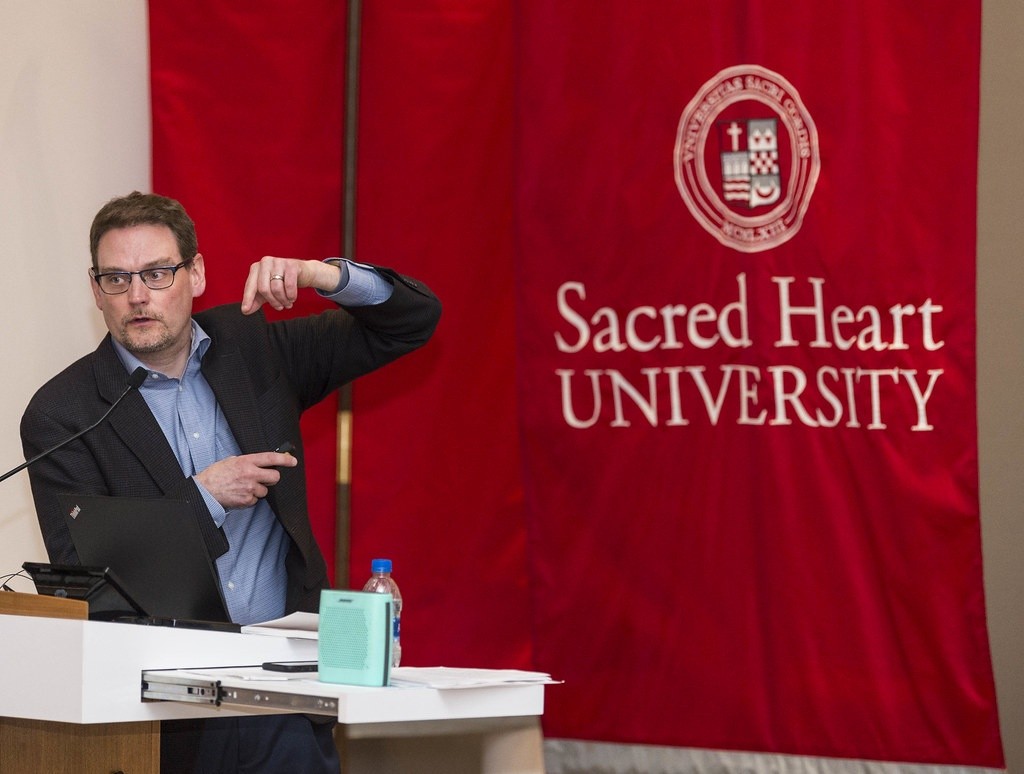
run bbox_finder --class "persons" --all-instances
[20,191,441,774]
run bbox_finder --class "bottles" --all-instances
[362,558,402,668]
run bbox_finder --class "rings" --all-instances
[270,274,284,280]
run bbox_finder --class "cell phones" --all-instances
[261,659,319,673]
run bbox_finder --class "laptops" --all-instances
[56,493,233,623]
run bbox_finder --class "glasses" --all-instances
[91,257,193,295]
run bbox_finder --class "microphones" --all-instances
[0,366,149,483]
